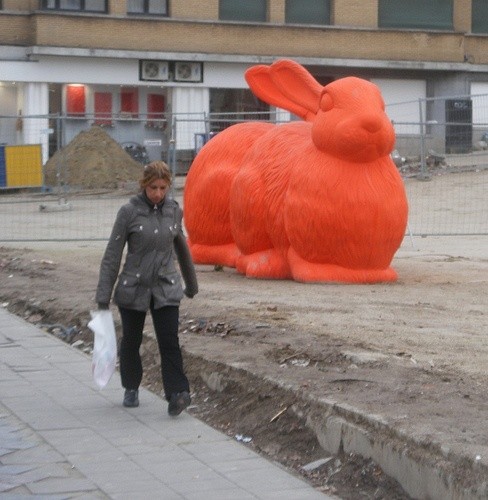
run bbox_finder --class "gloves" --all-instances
[98,301,109,312]
[182,288,193,299]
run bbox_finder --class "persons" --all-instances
[91,160,199,417]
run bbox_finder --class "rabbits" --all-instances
[182,59,410,285]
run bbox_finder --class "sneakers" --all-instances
[122,387,141,407]
[166,390,193,417]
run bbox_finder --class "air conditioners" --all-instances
[173,60,205,83]
[139,60,171,82]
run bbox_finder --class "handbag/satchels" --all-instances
[86,307,116,392]
